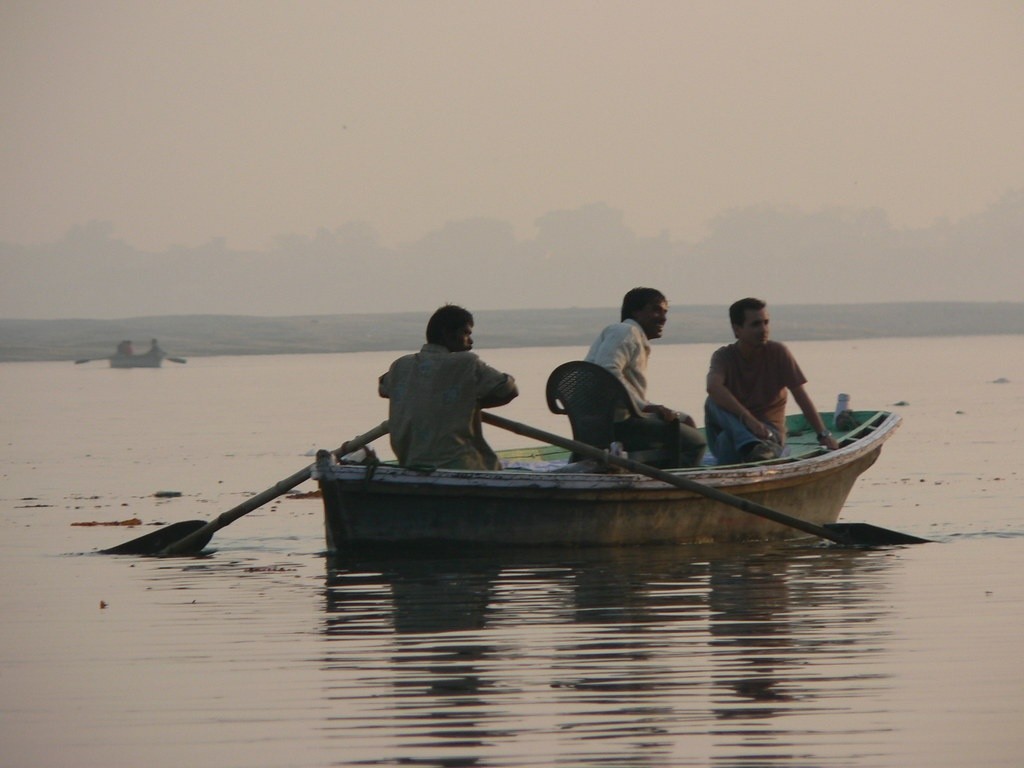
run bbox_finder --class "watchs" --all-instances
[816,430,834,441]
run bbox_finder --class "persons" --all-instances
[706,297,838,462]
[583,286,706,468]
[378,303,520,473]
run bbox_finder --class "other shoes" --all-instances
[749,443,777,462]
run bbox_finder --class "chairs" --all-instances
[546,361,682,470]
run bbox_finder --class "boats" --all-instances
[314,405,907,553]
[110,350,166,368]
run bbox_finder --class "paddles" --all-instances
[162,356,187,364]
[74,355,110,364]
[482,407,928,550]
[98,423,393,558]
